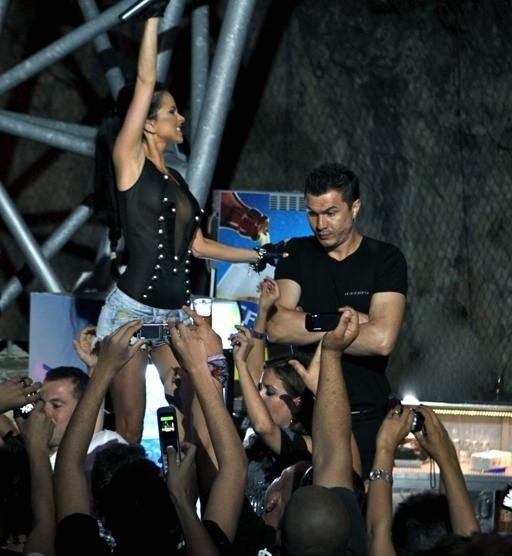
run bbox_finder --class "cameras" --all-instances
[137,322,172,342]
[399,409,422,432]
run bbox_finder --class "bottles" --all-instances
[220,191,269,242]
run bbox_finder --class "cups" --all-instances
[192,298,213,330]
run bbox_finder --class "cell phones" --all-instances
[18,394,36,420]
[302,310,343,333]
[156,405,181,477]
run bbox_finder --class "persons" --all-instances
[262,159,408,517]
[89,0,266,521]
[0,273,512,555]
